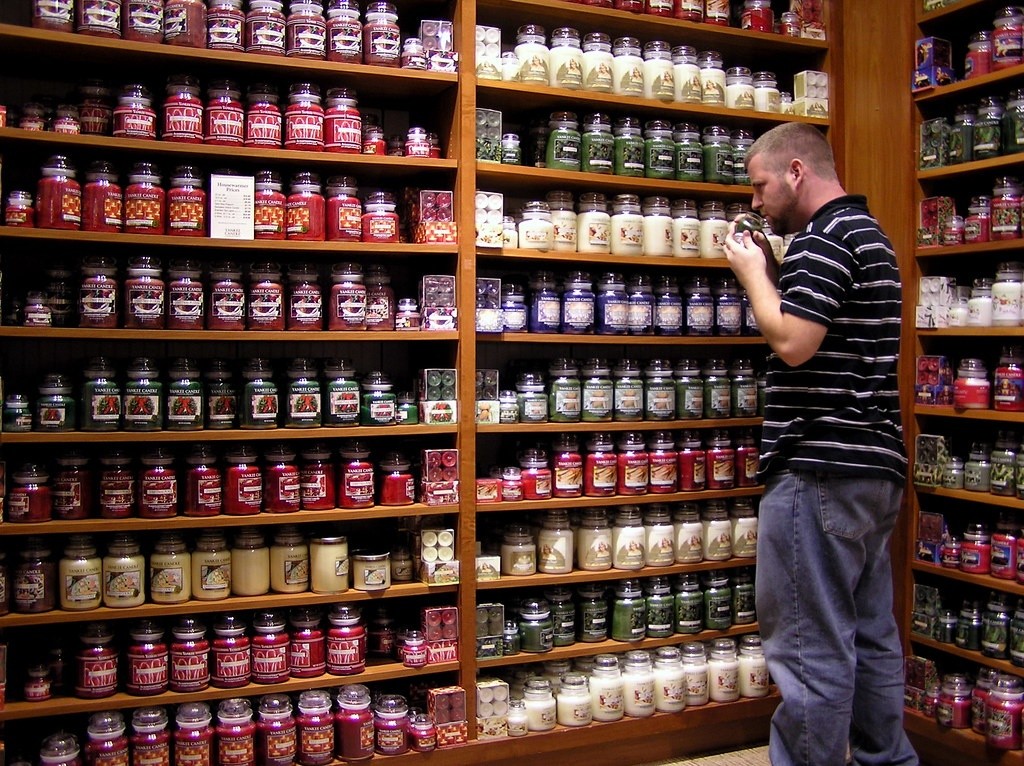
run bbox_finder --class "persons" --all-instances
[723,122,919,766]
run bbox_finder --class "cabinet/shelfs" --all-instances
[0,0,872,766]
[872,0,1024,766]
